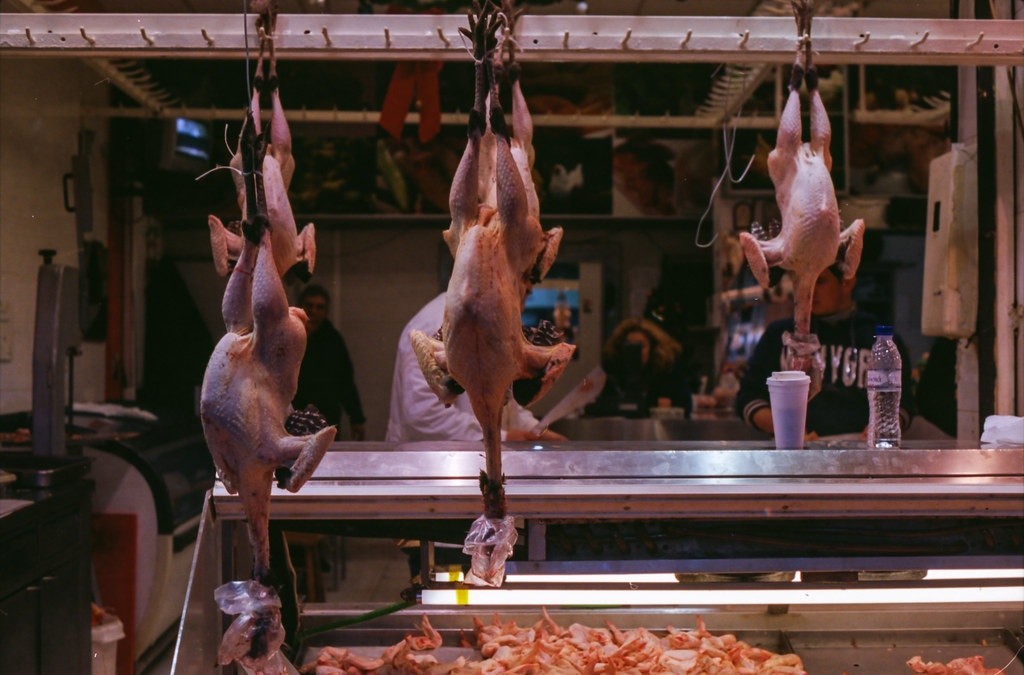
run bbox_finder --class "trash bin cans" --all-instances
[91,620,125,675]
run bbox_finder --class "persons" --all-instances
[385,292,570,441]
[293,285,366,441]
[586,317,683,418]
[735,262,915,442]
[548,524,658,556]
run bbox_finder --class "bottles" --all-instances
[866,325,902,449]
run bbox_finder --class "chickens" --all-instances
[411,0,575,558]
[207,5,315,284]
[738,0,864,399]
[199,109,338,660]
[549,164,583,196]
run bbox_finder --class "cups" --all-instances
[766,370,811,449]
